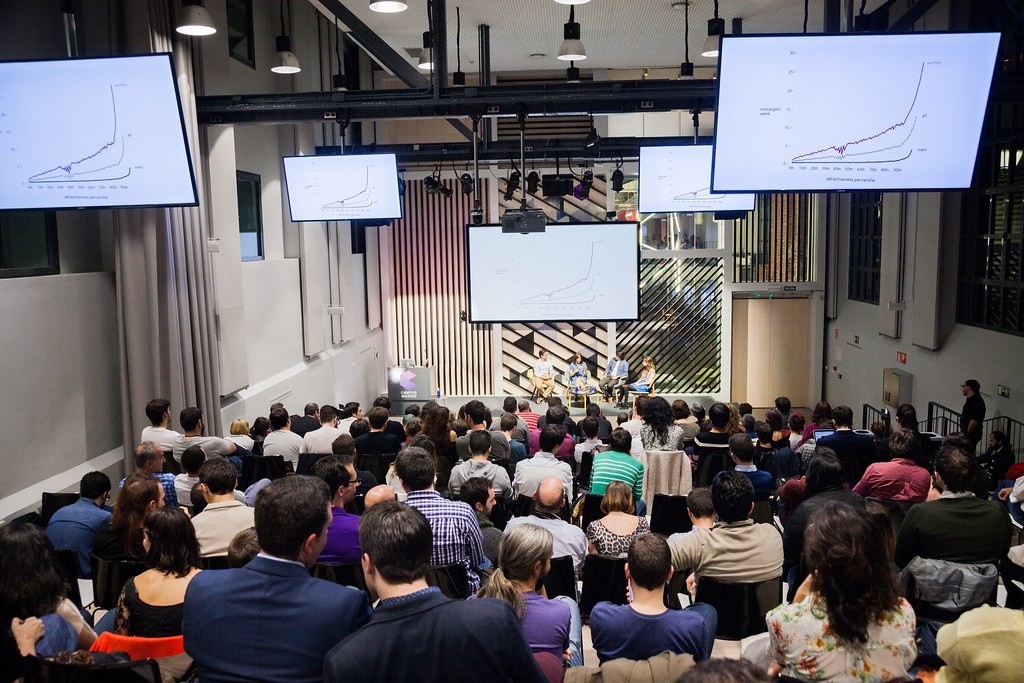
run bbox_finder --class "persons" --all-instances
[654,232,705,267]
[569,352,590,405]
[598,352,629,403]
[0,393,1024,683]
[959,378,986,456]
[613,356,655,410]
[532,348,556,404]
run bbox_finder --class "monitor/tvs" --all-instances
[0,52,200,214]
[637,29,1004,214]
[281,152,403,223]
[401,359,415,366]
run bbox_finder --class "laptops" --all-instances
[813,429,836,443]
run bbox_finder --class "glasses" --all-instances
[350,477,362,487]
[106,493,111,501]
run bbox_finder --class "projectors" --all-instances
[501,208,545,234]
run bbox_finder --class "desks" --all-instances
[568,389,600,409]
[379,393,448,408]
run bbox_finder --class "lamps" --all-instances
[565,60,581,83]
[610,158,625,193]
[470,196,485,224]
[421,164,454,199]
[397,169,407,195]
[456,160,473,195]
[369,0,408,13]
[418,31,434,70]
[270,0,302,74]
[175,0,218,36]
[580,116,602,150]
[852,0,873,32]
[524,158,541,195]
[556,5,587,61]
[701,0,725,57]
[450,6,467,87]
[887,300,908,312]
[577,164,594,200]
[500,158,521,201]
[670,0,703,113]
[332,13,348,91]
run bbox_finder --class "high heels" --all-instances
[619,403,630,409]
[613,401,623,409]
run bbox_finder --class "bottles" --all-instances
[437,388,440,397]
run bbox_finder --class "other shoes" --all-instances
[602,397,607,402]
[608,396,613,404]
[536,396,543,404]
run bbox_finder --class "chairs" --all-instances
[601,373,625,403]
[631,383,655,410]
[527,370,554,403]
[0,438,1024,683]
[566,370,591,398]
